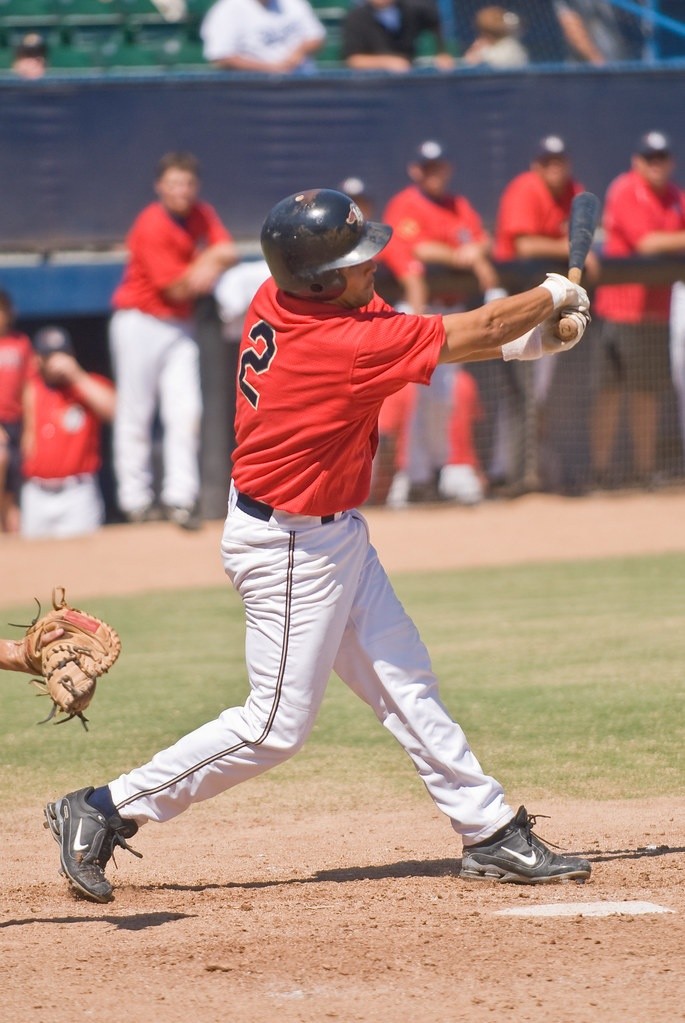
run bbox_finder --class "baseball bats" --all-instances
[553,191,604,340]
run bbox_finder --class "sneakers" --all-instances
[460,805,591,884]
[46,784,144,903]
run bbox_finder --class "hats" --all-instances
[411,141,450,168]
[35,329,72,355]
[532,137,567,163]
[634,132,672,157]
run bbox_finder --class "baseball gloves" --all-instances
[24,606,121,714]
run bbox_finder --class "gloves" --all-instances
[545,272,590,313]
[503,309,591,368]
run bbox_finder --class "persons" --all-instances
[337,139,507,506]
[583,130,685,489]
[43,189,593,906]
[486,136,602,486]
[0,584,121,730]
[341,1,624,71]
[109,155,235,524]
[0,289,36,531]
[16,326,115,537]
[198,0,327,74]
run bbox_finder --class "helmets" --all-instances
[260,187,394,301]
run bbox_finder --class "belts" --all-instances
[235,491,335,525]
